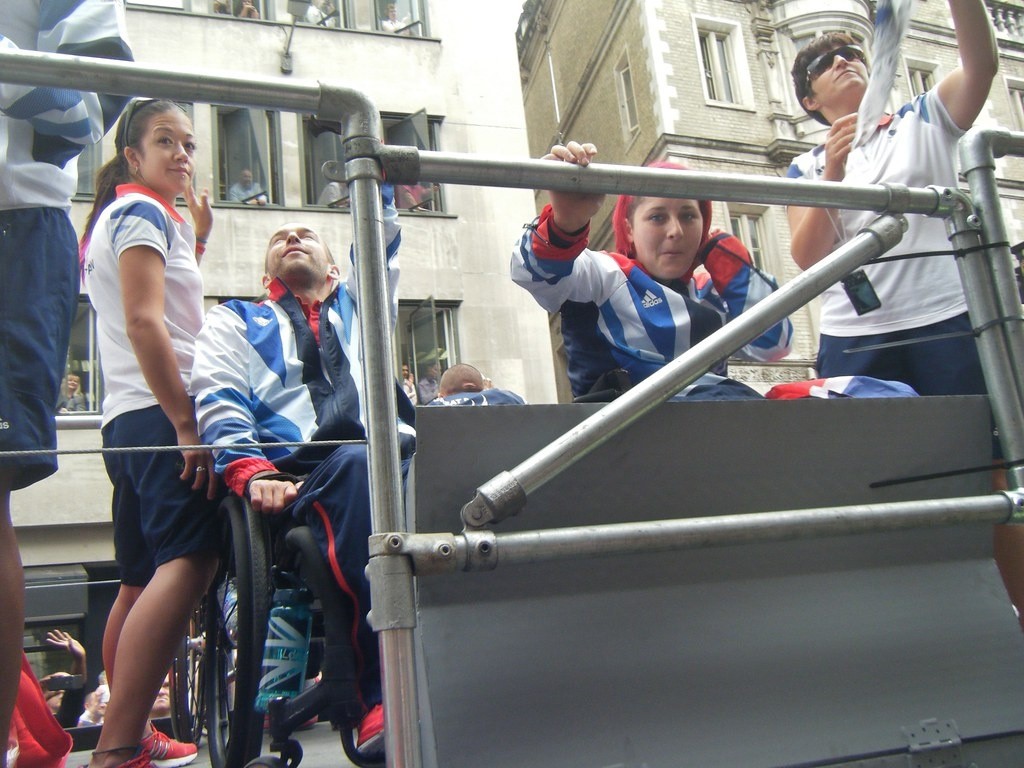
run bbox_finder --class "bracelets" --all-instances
[194,237,208,244]
[195,243,206,254]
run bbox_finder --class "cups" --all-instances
[256,588,312,710]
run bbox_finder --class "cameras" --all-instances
[842,270,881,316]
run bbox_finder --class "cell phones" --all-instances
[49,674,82,690]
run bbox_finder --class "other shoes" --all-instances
[356,704,386,756]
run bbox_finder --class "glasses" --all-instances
[805,45,867,95]
[123,97,187,147]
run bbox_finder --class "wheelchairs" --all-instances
[203,491,390,768]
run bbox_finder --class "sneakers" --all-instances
[78,747,157,768]
[141,722,197,768]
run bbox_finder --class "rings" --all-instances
[196,466,207,472]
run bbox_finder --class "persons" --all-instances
[425,364,526,407]
[510,139,796,401]
[0,0,136,767]
[420,362,440,404]
[77,93,230,766]
[401,362,418,407]
[786,0,1024,630]
[186,172,420,757]
[55,374,87,413]
[214,0,412,36]
[226,168,439,213]
[36,572,244,729]
